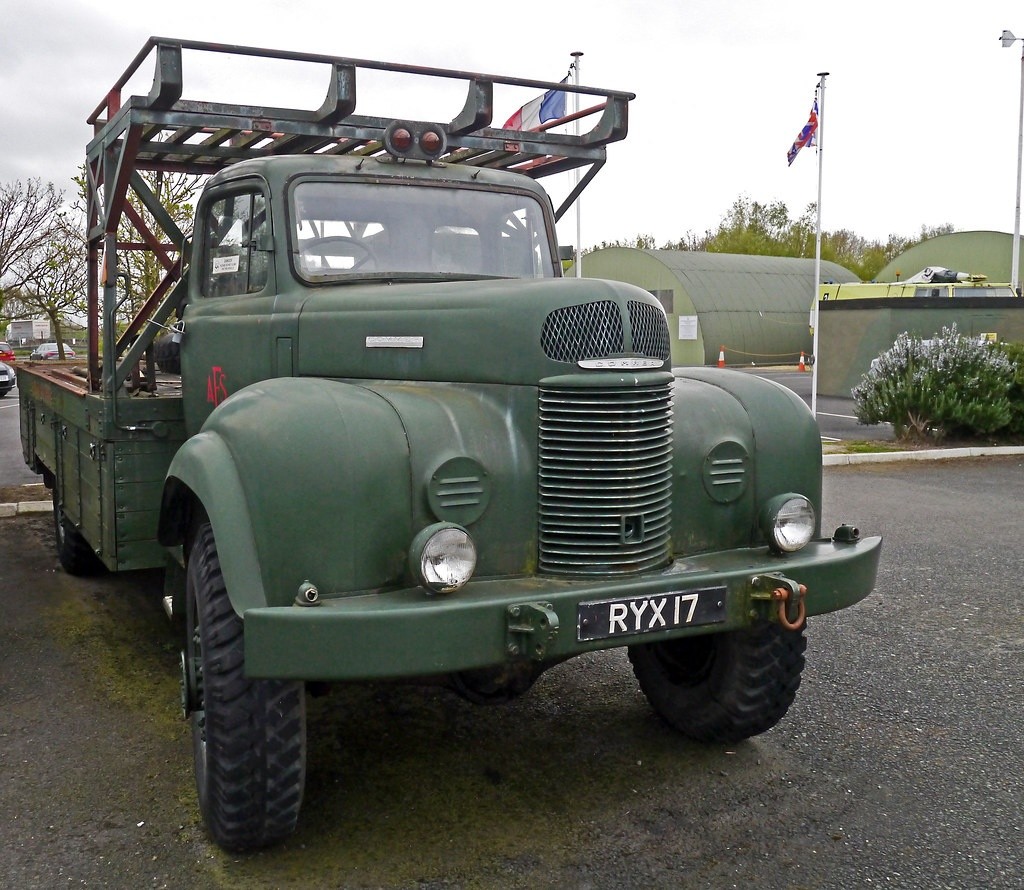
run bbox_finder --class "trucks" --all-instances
[16,33,881,859]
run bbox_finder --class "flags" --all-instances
[502,74,566,170]
[786,90,819,167]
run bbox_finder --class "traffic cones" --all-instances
[716,346,726,368]
[796,351,806,372]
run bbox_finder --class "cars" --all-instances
[0,361,16,397]
[7,339,18,345]
[29,343,75,360]
[0,341,16,362]
